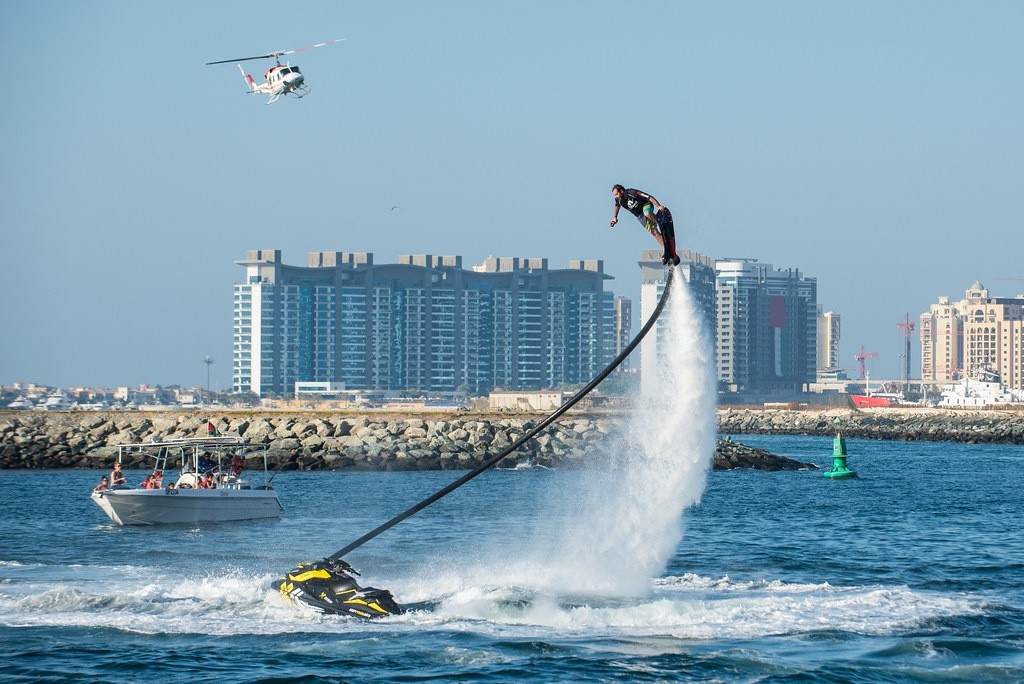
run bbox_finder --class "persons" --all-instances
[610,184,666,260]
[141,470,162,489]
[197,452,219,474]
[202,472,215,489]
[94,476,110,491]
[168,482,175,490]
[181,455,196,473]
[193,476,203,489]
[110,461,127,489]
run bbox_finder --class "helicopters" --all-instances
[204,34,348,104]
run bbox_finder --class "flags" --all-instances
[208,422,219,437]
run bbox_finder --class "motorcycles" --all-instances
[270,555,401,623]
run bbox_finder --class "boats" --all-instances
[90,434,297,526]
[935,359,1024,405]
[851,382,929,408]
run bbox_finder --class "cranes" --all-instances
[855,344,878,383]
[896,312,965,379]
